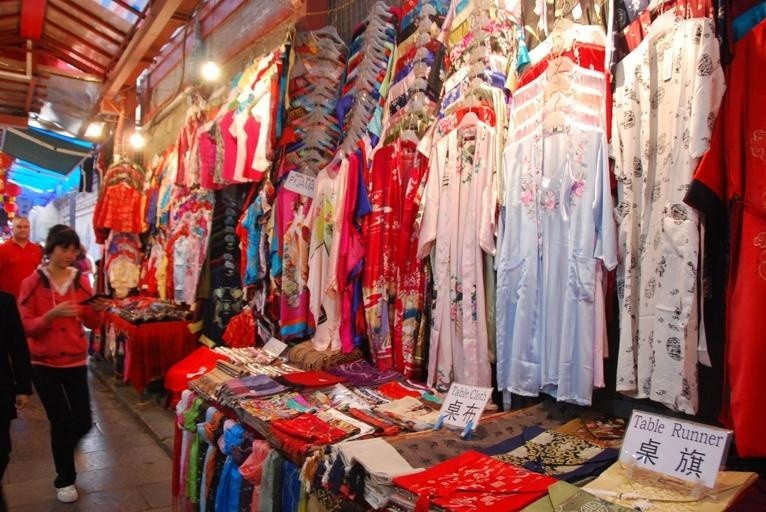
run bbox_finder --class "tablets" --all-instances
[80,294,113,304]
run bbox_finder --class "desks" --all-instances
[86,292,202,406]
[161,336,766,511]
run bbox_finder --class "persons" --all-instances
[91,257,101,289]
[71,241,93,287]
[1,291,34,502]
[0,213,43,302]
[15,223,108,504]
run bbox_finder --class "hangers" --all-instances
[275,0,720,183]
[106,47,281,189]
[204,189,249,296]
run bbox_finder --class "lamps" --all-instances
[126,68,147,153]
[198,0,224,84]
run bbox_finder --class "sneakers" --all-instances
[55,484,79,503]
